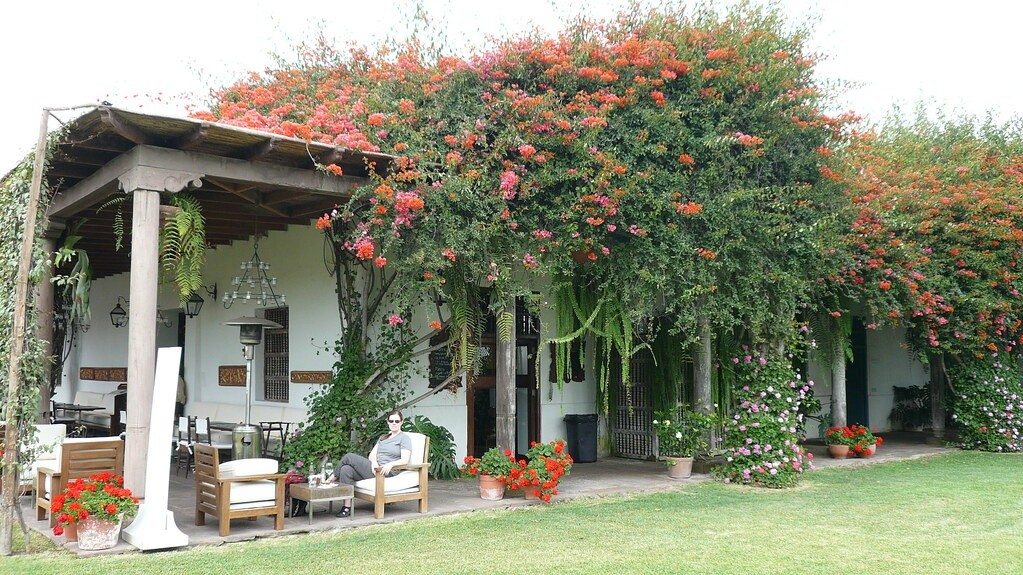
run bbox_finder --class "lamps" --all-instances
[110,296,129,328]
[222,233,287,312]
[180,275,217,319]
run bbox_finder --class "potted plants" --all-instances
[889,380,933,432]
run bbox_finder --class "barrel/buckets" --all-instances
[231,424,263,460]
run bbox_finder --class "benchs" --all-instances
[51,391,117,437]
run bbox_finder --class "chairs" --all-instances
[37,436,124,529]
[18,424,66,494]
[175,414,216,479]
[193,443,285,536]
[353,431,431,519]
[194,416,232,466]
[49,400,75,438]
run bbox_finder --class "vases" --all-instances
[829,444,851,459]
[664,457,694,478]
[77,511,126,551]
[504,484,525,497]
[64,523,77,542]
[523,483,542,500]
[479,475,505,500]
[856,444,876,459]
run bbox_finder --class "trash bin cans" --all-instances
[232,425,264,459]
[563,412,599,463]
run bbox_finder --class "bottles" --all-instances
[309,462,314,475]
[320,466,325,484]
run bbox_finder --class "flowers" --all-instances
[823,423,883,456]
[50,471,140,536]
[462,437,574,503]
[652,397,720,468]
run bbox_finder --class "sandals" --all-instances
[336,506,351,517]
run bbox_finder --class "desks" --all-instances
[289,483,354,524]
[190,422,283,463]
[55,404,106,436]
[174,416,195,466]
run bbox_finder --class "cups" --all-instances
[309,476,316,487]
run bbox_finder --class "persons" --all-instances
[174,374,188,424]
[325,410,413,517]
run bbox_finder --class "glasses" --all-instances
[388,420,400,423]
